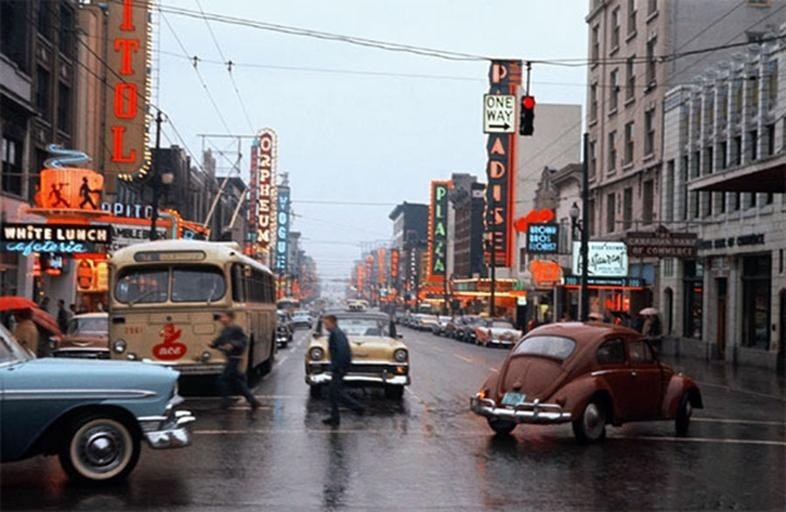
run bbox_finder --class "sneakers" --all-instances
[320,414,342,426]
[217,396,262,416]
[356,405,367,416]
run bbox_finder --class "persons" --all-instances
[544,308,550,323]
[321,315,366,424]
[0,297,107,362]
[48,182,70,207]
[591,317,597,321]
[561,309,571,322]
[79,178,98,210]
[606,311,652,336]
[528,316,536,332]
[210,311,262,417]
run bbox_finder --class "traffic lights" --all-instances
[521,95,535,136]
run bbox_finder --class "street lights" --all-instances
[151,110,174,242]
[571,133,589,323]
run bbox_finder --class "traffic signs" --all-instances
[483,94,518,137]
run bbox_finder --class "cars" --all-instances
[304,308,412,403]
[469,320,704,445]
[48,312,110,361]
[274,294,523,350]
[0,321,194,489]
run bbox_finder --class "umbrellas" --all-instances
[588,312,604,320]
[640,307,659,315]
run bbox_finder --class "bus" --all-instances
[108,240,276,397]
